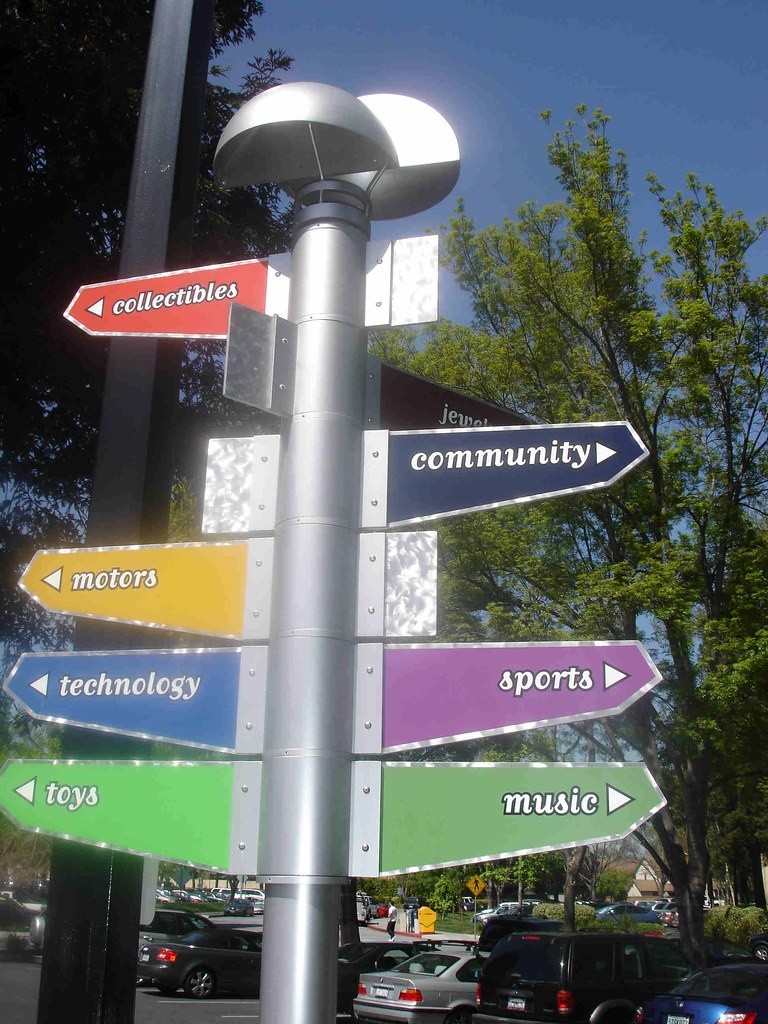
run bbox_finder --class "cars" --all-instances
[337,941,441,994]
[473,899,565,951]
[634,964,768,1024]
[749,933,768,961]
[354,946,492,1024]
[0,898,42,950]
[137,929,263,999]
[155,881,265,916]
[573,895,724,929]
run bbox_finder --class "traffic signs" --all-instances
[63,230,439,339]
[202,302,650,534]
[0,760,668,877]
[2,640,664,755]
[17,531,438,640]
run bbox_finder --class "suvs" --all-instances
[471,935,768,1024]
[30,908,216,986]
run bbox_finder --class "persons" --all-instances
[386,901,398,943]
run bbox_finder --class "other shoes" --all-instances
[388,935,396,942]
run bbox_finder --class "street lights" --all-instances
[212,83,460,1024]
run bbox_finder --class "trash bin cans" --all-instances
[417,907,436,935]
